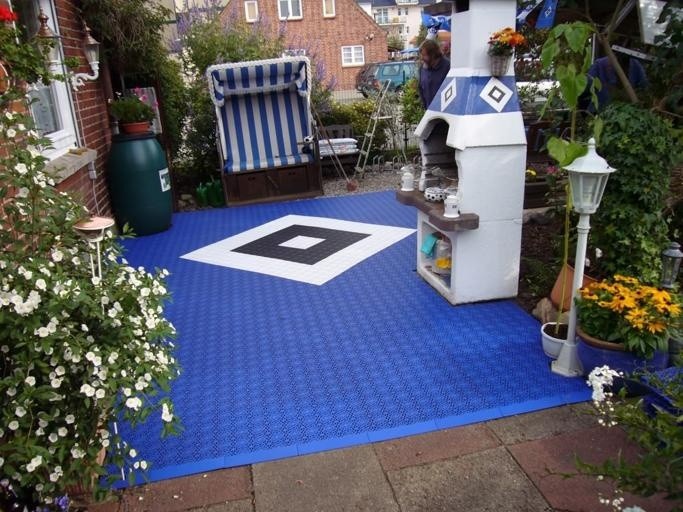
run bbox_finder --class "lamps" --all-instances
[27,5,102,91]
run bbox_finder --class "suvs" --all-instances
[359,60,424,103]
[354,61,389,99]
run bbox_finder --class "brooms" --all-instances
[311,102,358,192]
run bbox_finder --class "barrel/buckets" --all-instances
[105,131,173,238]
[431,240,452,275]
[576,341,670,381]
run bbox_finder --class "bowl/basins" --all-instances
[424,189,446,202]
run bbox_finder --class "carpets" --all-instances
[66,191,672,499]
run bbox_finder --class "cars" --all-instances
[512,45,556,80]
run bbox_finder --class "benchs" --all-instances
[313,125,360,174]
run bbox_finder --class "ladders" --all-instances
[351,78,408,182]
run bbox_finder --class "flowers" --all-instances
[574,274,679,345]
[108,87,160,121]
[573,239,611,274]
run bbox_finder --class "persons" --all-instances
[588,36,648,114]
[416,41,450,111]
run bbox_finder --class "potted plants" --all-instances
[541,23,581,358]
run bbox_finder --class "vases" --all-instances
[549,259,601,313]
[117,122,151,136]
[576,324,671,376]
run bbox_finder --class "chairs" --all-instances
[208,56,327,209]
